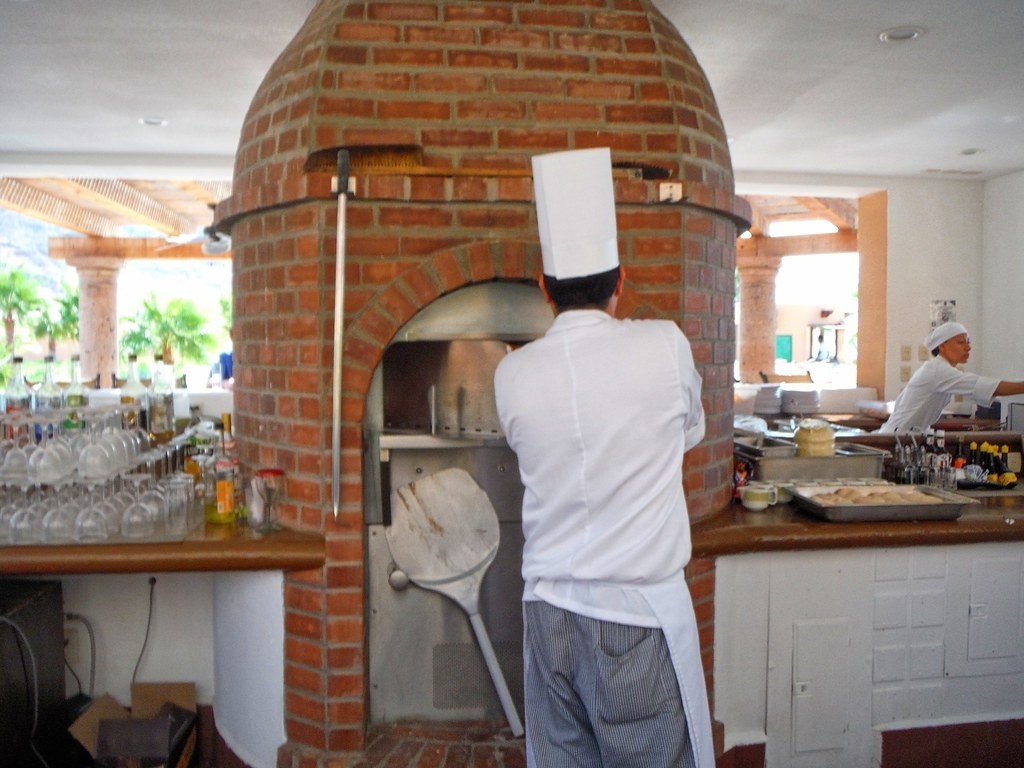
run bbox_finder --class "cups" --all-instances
[166,474,195,535]
[766,479,796,503]
[211,363,221,392]
[746,480,774,488]
[737,486,777,511]
[789,477,896,487]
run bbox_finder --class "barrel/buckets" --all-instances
[733,382,755,416]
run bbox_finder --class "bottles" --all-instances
[204,423,236,523]
[952,435,967,468]
[969,441,1018,488]
[4,356,32,442]
[149,354,174,442]
[35,356,63,438]
[218,413,244,514]
[64,354,87,432]
[121,354,148,430]
[885,428,952,490]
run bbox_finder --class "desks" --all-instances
[1,517,324,576]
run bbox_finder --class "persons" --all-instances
[816,336,829,361]
[878,322,1024,434]
[494,147,716,768]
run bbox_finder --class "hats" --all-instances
[923,322,967,353]
[531,145,620,280]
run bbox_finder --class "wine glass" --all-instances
[256,475,283,532]
[0,388,188,539]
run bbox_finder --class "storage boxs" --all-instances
[67,681,196,768]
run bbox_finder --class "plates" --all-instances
[753,383,781,413]
[781,383,820,413]
